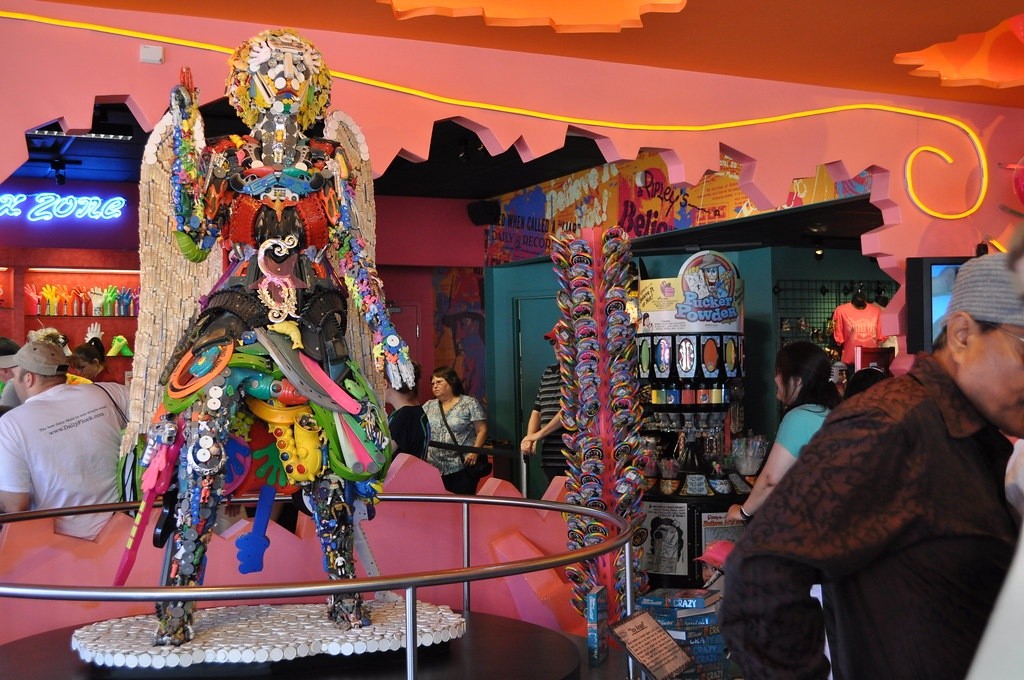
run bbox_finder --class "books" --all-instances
[636,589,729,680]
[587,586,609,667]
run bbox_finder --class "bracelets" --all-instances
[739,506,751,520]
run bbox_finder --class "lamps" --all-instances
[814,238,824,255]
[55,169,66,185]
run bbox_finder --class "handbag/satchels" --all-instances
[464,457,492,481]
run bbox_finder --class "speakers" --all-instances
[468,200,501,226]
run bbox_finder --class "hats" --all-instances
[693,539,736,568]
[543,322,559,341]
[0,341,70,377]
[943,252,1024,324]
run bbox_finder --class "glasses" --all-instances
[431,380,445,386]
[549,340,556,346]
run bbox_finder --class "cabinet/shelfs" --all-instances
[24,314,138,358]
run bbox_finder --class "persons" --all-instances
[0,328,130,541]
[832,292,883,366]
[693,540,735,583]
[719,222,1024,680]
[727,342,844,522]
[422,367,488,494]
[383,361,430,462]
[521,319,568,480]
